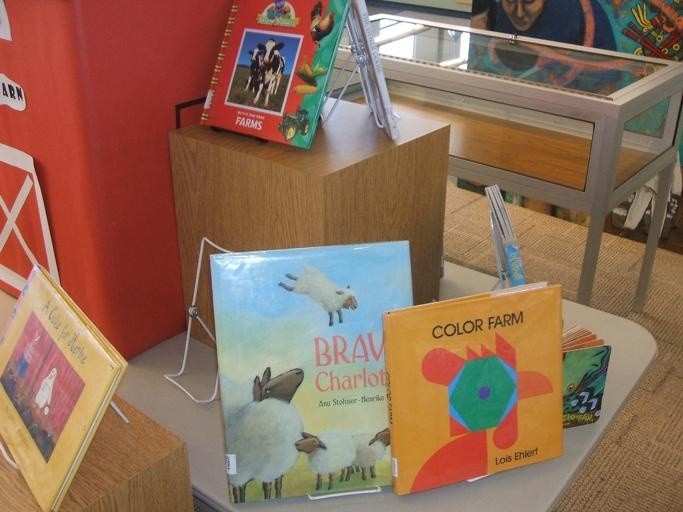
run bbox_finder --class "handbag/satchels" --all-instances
[382,280,564,496]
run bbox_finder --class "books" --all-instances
[199,1,354,151]
[1,263,129,512]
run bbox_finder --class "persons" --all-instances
[482,0,620,96]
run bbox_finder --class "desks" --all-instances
[111,261,660,512]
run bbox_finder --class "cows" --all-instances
[244,38,286,107]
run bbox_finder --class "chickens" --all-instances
[309,1,335,42]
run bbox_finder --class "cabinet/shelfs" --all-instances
[331,12,681,304]
